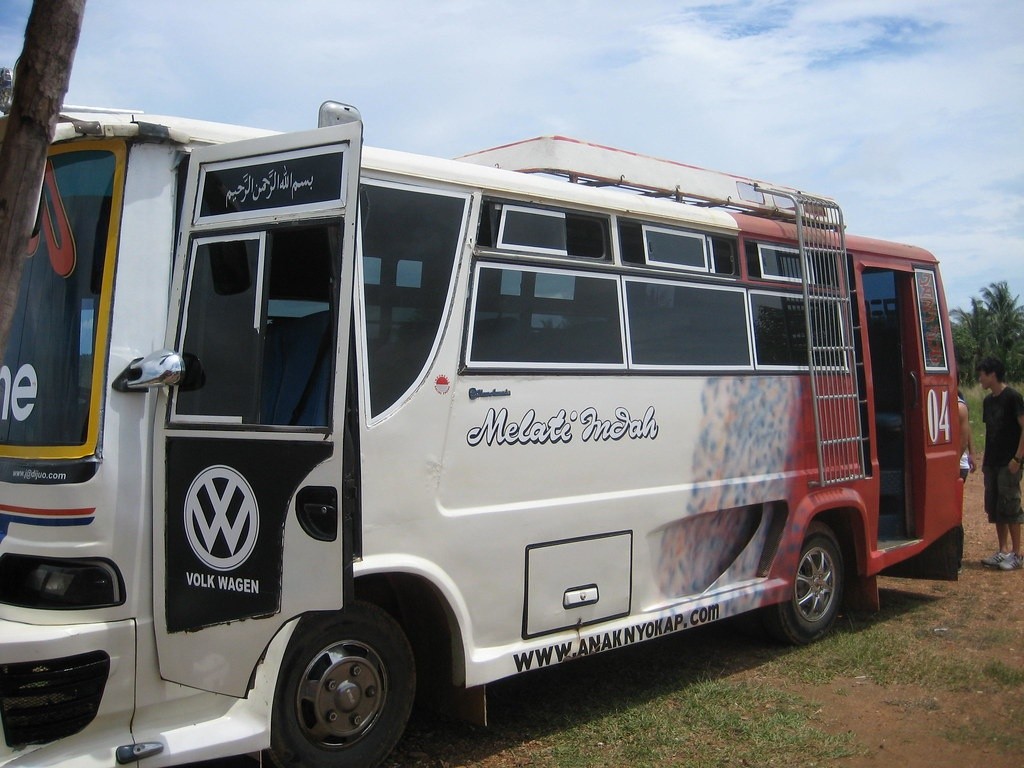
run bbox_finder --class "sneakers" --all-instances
[982,552,1013,564]
[999,554,1023,570]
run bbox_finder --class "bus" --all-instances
[0,69,969,768]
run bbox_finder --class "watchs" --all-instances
[1014,456,1022,463]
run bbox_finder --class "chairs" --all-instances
[853,297,903,436]
[262,310,358,484]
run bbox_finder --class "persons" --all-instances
[955,361,977,485]
[975,357,1024,572]
